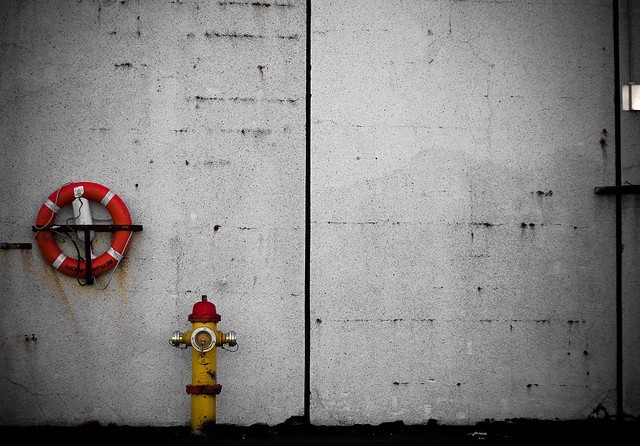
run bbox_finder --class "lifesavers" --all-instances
[35,182,132,276]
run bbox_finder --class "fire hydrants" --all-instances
[168,295,239,438]
